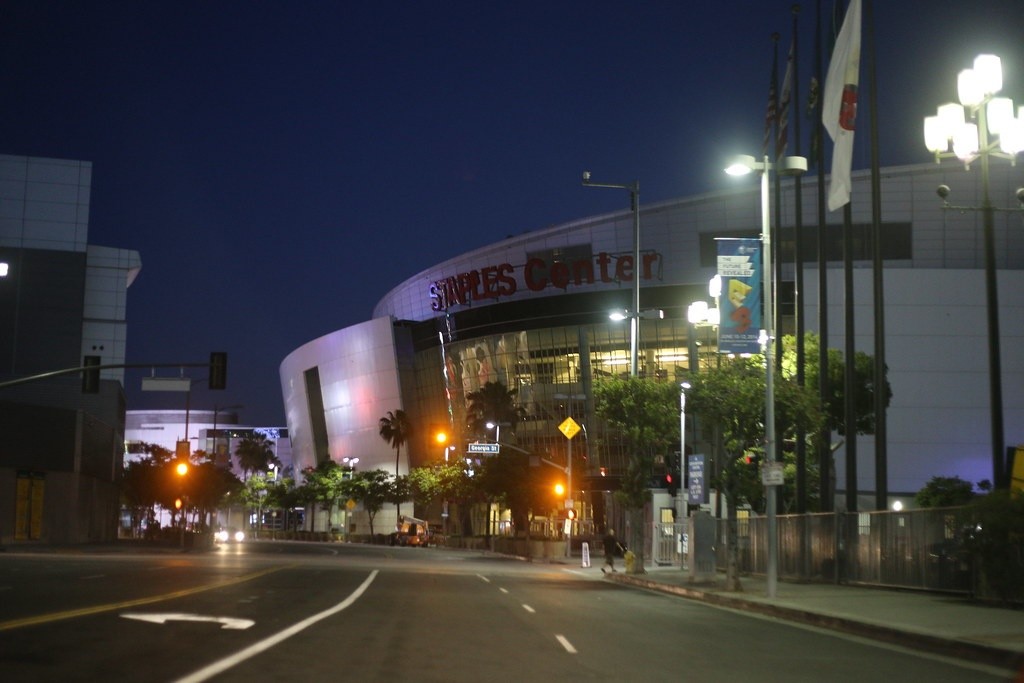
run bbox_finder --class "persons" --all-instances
[600,528,623,573]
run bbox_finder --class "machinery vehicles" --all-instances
[388,514,430,547]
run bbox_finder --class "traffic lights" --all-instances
[176,497,184,510]
[483,421,500,457]
[549,480,569,501]
[427,421,452,449]
[665,454,676,497]
[557,508,580,522]
[308,466,315,474]
[176,462,194,478]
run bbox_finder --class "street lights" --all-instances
[580,171,665,381]
[681,381,692,522]
[722,150,809,599]
[342,458,360,481]
[924,52,1024,507]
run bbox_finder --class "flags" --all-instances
[760,20,821,169]
[823,0,861,212]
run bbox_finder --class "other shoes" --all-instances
[601,568,605,573]
